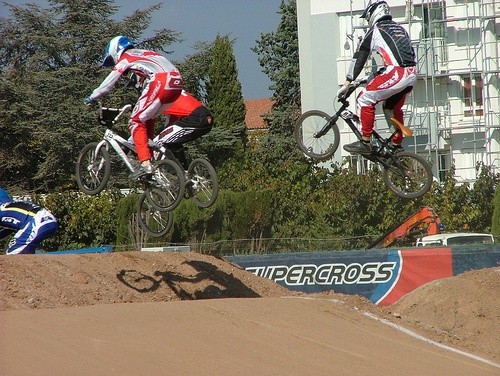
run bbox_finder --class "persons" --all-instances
[127,74,213,171]
[337,0,418,154]
[0,188,58,254]
[83,36,184,179]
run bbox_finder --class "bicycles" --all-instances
[75,99,187,213]
[123,136,219,238]
[294,76,434,200]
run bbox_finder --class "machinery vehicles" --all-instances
[365,206,443,249]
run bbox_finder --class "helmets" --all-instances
[101,35,135,65]
[360,0,392,27]
[0,187,11,205]
[126,72,148,96]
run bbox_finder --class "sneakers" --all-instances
[343,140,373,155]
[389,144,405,154]
[128,164,152,180]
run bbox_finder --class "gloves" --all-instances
[361,73,375,84]
[83,96,95,108]
[335,82,348,100]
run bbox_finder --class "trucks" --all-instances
[415,233,494,247]
